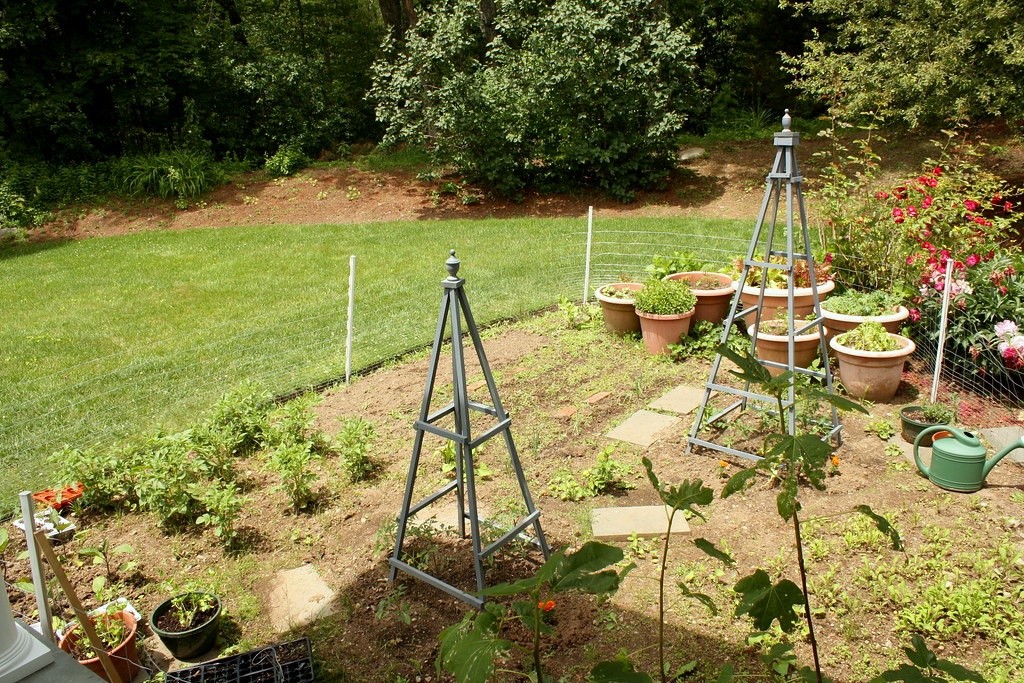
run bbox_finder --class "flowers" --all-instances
[732,250,838,291]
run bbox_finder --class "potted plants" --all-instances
[595,282,647,336]
[57,603,141,683]
[830,320,916,402]
[899,396,958,447]
[814,291,910,364]
[632,270,699,354]
[662,271,735,335]
[149,567,221,660]
[745,305,826,377]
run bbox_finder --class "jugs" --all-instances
[913,425,1024,492]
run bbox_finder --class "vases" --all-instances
[730,277,835,335]
